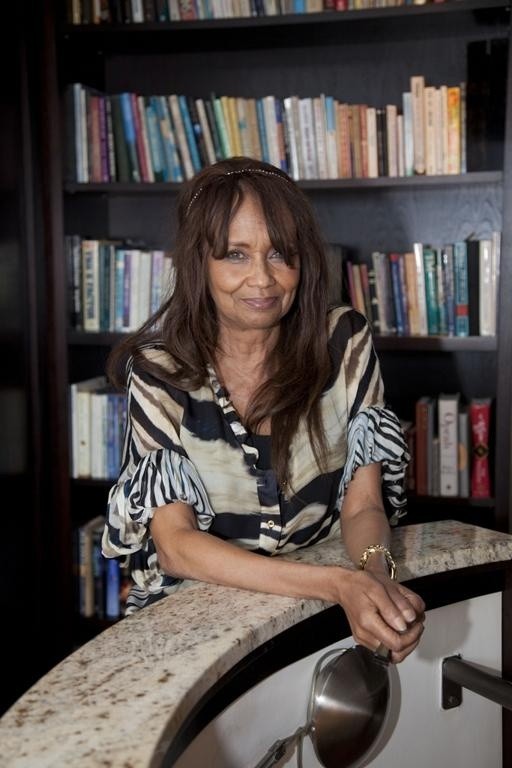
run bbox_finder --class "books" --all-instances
[346,231,500,336]
[403,391,492,498]
[70,234,176,332]
[81,518,131,618]
[74,76,468,184]
[74,0,424,25]
[71,377,124,478]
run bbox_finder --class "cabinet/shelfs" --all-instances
[55,1,511,629]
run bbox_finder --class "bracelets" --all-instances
[357,545,396,580]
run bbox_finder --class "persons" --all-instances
[101,158,425,663]
